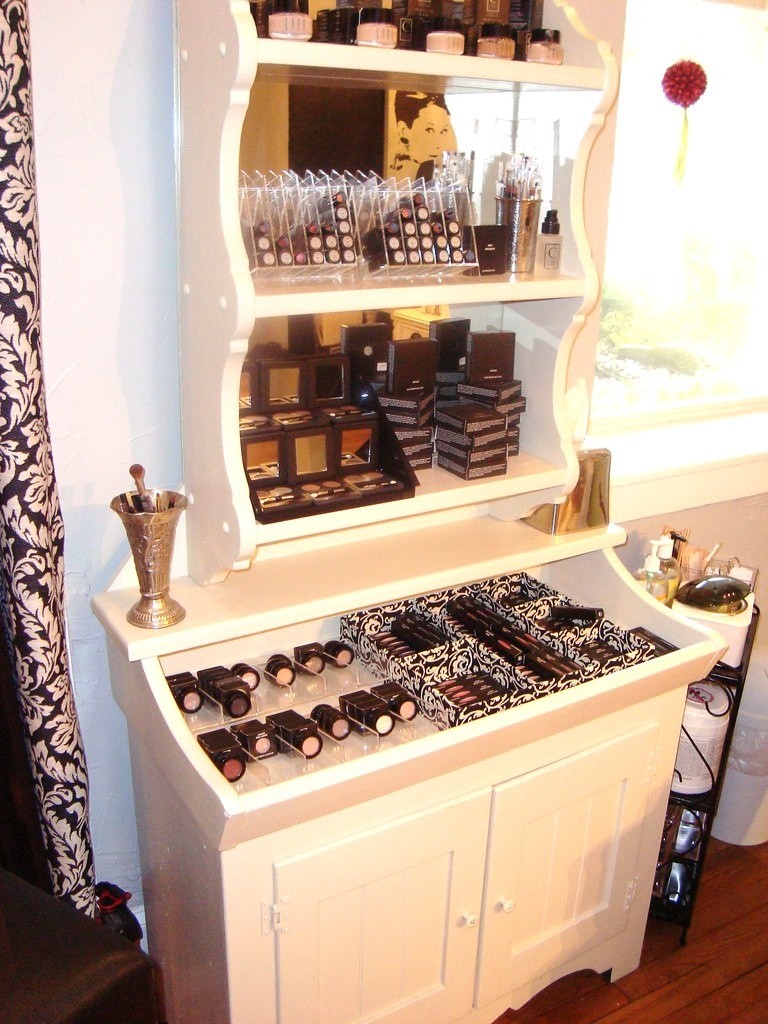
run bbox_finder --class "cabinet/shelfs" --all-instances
[89,0,730,1024]
